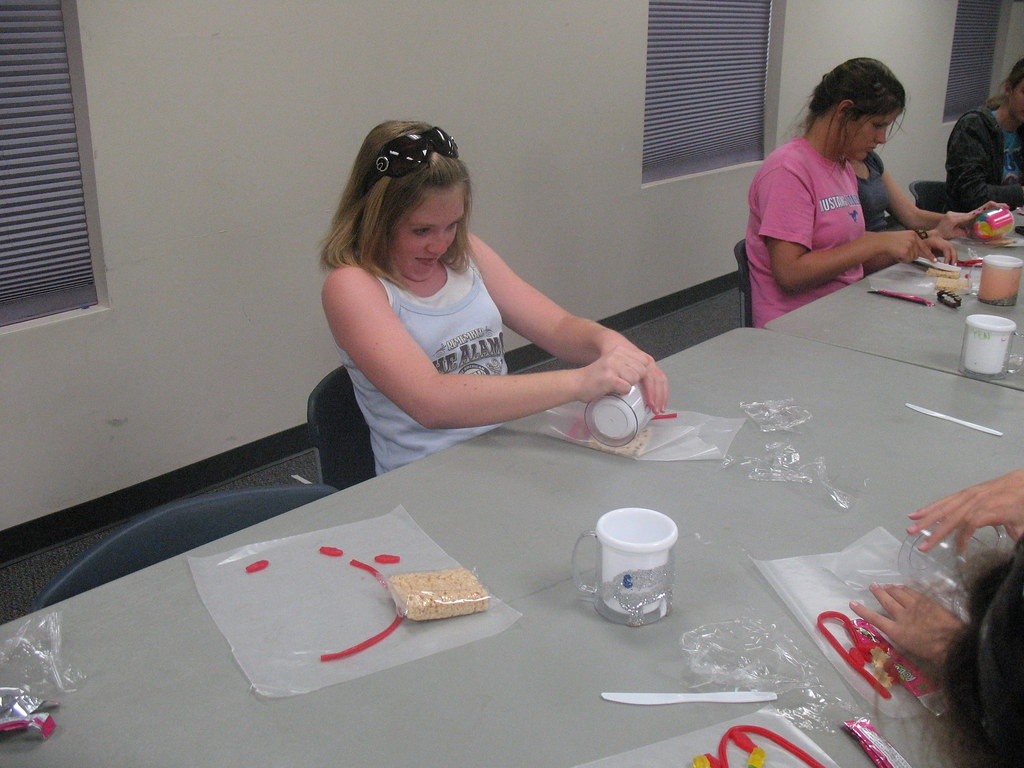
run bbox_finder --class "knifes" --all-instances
[601,692,777,705]
[915,257,961,272]
[905,403,1003,436]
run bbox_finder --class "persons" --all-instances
[849,468,1024,768]
[745,57,1009,329]
[321,120,670,476]
[942,56,1024,213]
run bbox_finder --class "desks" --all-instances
[0,235,1024,768]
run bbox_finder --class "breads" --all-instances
[988,238,1016,245]
[588,425,652,457]
[926,267,969,291]
[390,570,489,620]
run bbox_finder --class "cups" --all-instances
[897,525,1006,617]
[968,255,1023,306]
[974,207,1015,240]
[957,314,1017,380]
[584,383,655,448]
[571,507,678,628]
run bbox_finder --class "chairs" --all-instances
[909,180,946,214]
[306,366,376,490]
[32,486,340,612]
[734,238,752,327]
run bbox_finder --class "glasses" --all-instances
[354,126,459,202]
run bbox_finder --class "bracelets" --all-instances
[915,228,928,239]
[937,290,962,308]
